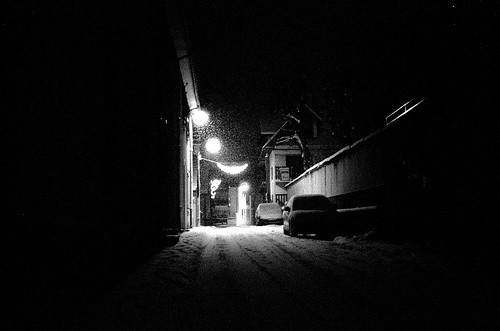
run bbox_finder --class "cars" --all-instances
[282,195,339,236]
[255,203,283,225]
